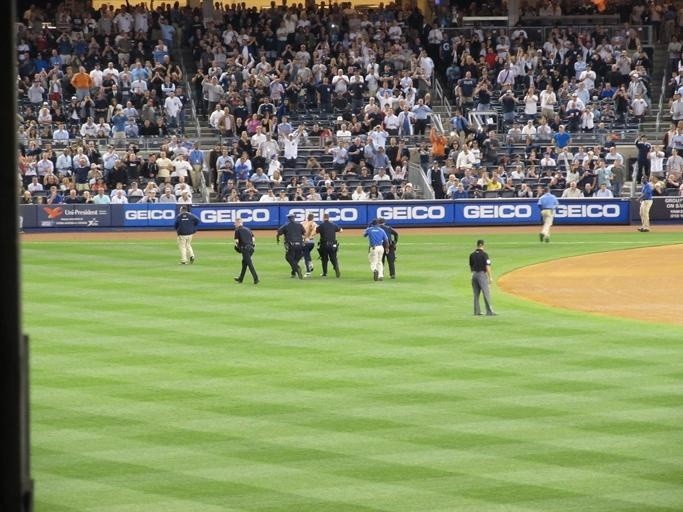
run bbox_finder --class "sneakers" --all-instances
[290,262,341,278]
[636,226,649,232]
[179,260,188,265]
[189,255,195,264]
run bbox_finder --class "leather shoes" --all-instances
[233,277,242,283]
[380,277,383,281]
[485,312,499,316]
[374,270,379,281]
[538,232,544,242]
[253,279,259,284]
[474,312,483,316]
[545,238,549,242]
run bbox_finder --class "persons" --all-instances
[277,213,343,280]
[363,217,398,280]
[14,0,683,203]
[174,206,201,266]
[638,176,653,233]
[233,218,259,283]
[538,186,558,242]
[466,238,500,316]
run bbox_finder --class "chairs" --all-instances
[10,5,681,204]
